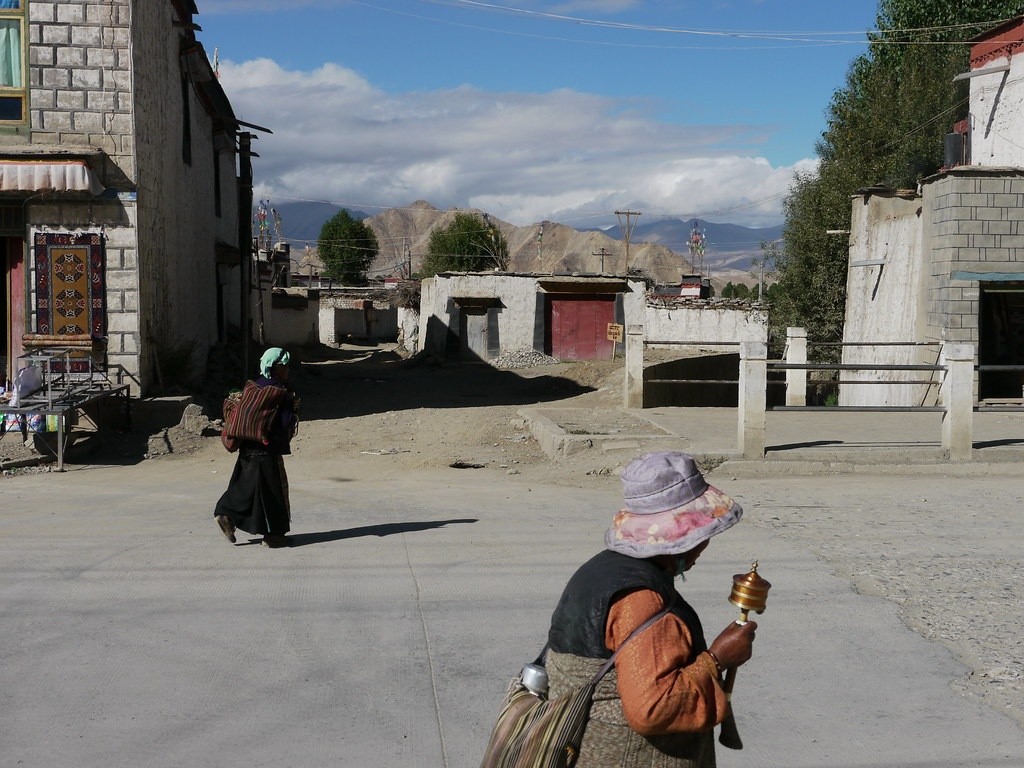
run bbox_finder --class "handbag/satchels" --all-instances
[479,593,678,767]
[222,389,242,452]
[226,379,286,444]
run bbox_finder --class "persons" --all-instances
[214,348,304,547]
[545,451,759,768]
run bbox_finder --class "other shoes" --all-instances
[263,535,294,546]
[217,515,237,544]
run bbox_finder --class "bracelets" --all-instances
[706,650,722,682]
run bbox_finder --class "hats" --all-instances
[604,450,743,560]
[259,347,293,378]
